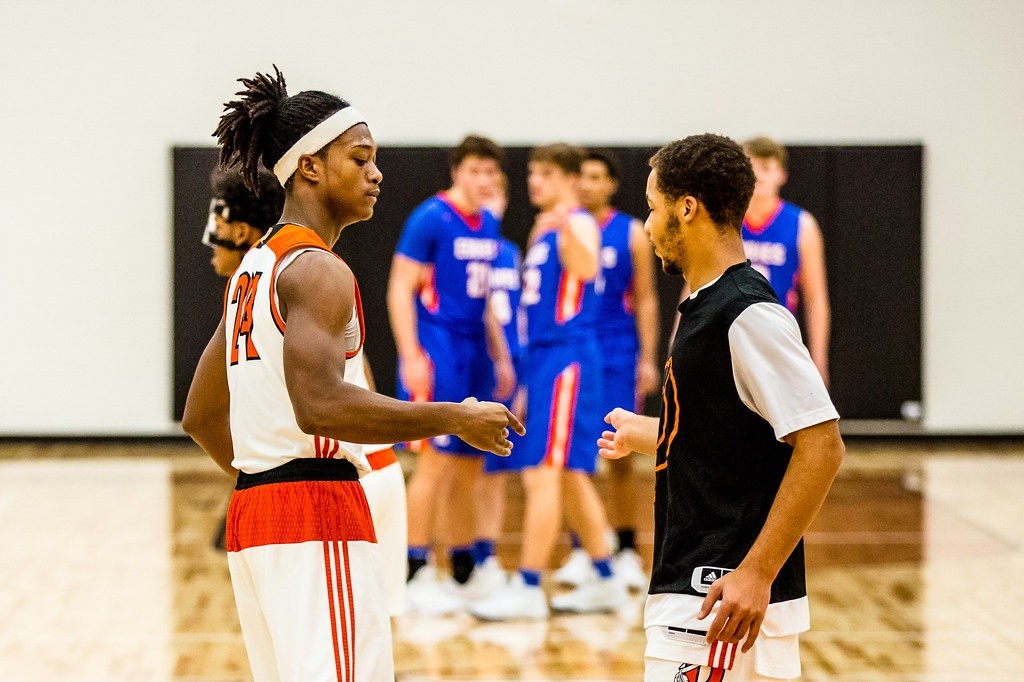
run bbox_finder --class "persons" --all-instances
[384,134,828,617]
[396,614,637,682]
[200,164,408,617]
[594,133,846,682]
[180,66,526,682]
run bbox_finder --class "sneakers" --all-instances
[470,572,549,618]
[619,548,647,587]
[551,550,592,584]
[549,569,628,614]
[464,556,507,595]
[404,564,457,615]
